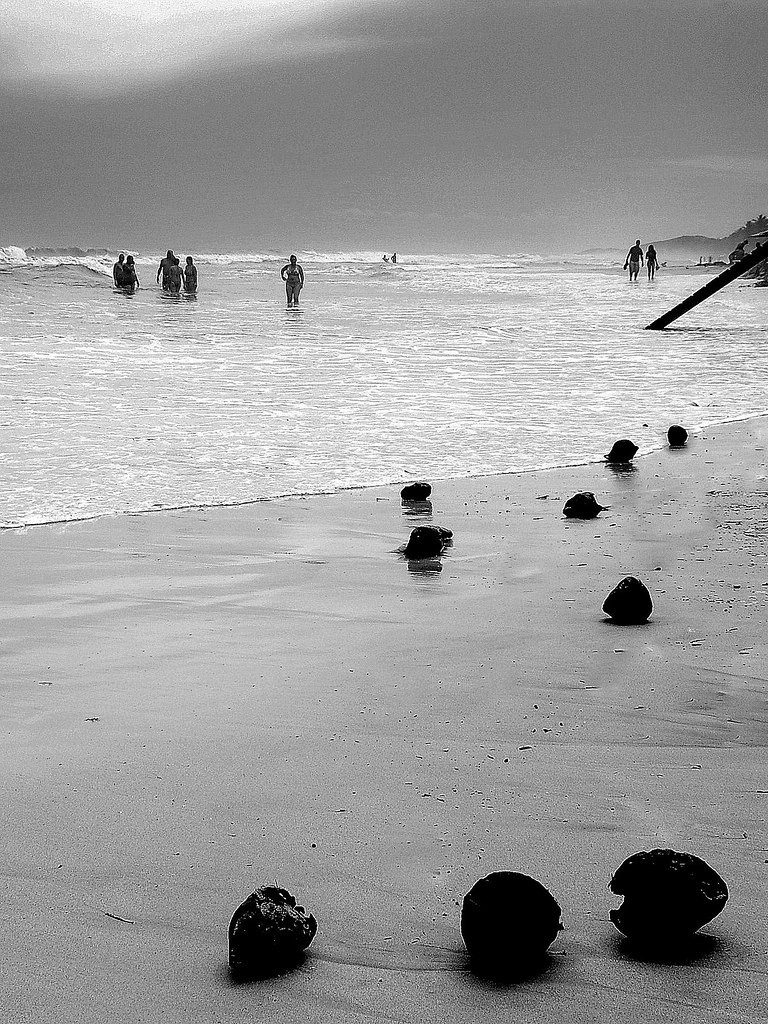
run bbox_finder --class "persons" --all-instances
[157,250,176,290]
[391,253,396,263]
[382,255,389,262]
[700,256,702,264]
[645,244,658,280]
[114,254,139,290]
[735,240,748,251]
[281,255,304,303]
[167,258,184,292]
[756,242,761,248]
[626,240,644,281]
[184,256,197,291]
